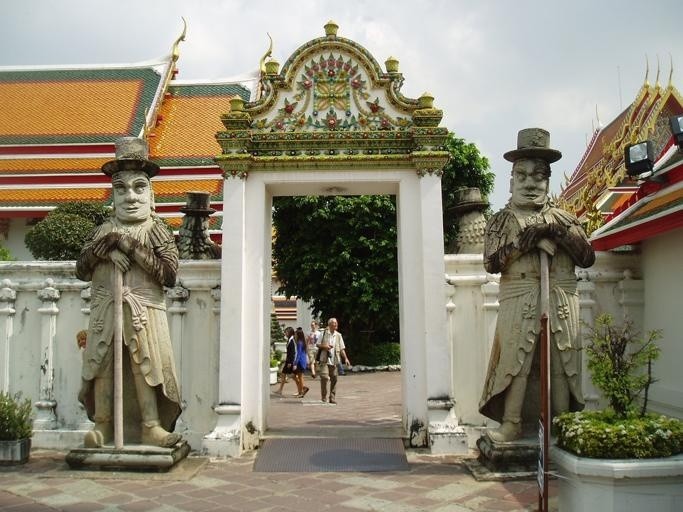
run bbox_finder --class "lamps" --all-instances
[668,114,682,154]
[622,140,665,186]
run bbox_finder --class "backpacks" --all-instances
[314,348,331,363]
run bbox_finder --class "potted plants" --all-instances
[268,358,278,384]
[0,389,35,464]
[548,309,683,512]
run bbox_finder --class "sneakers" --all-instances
[291,385,309,398]
[321,396,336,404]
[272,390,282,395]
[338,372,347,376]
[311,374,316,380]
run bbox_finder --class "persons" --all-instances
[316,317,350,404]
[477,129,595,445]
[272,320,325,398]
[75,137,184,449]
